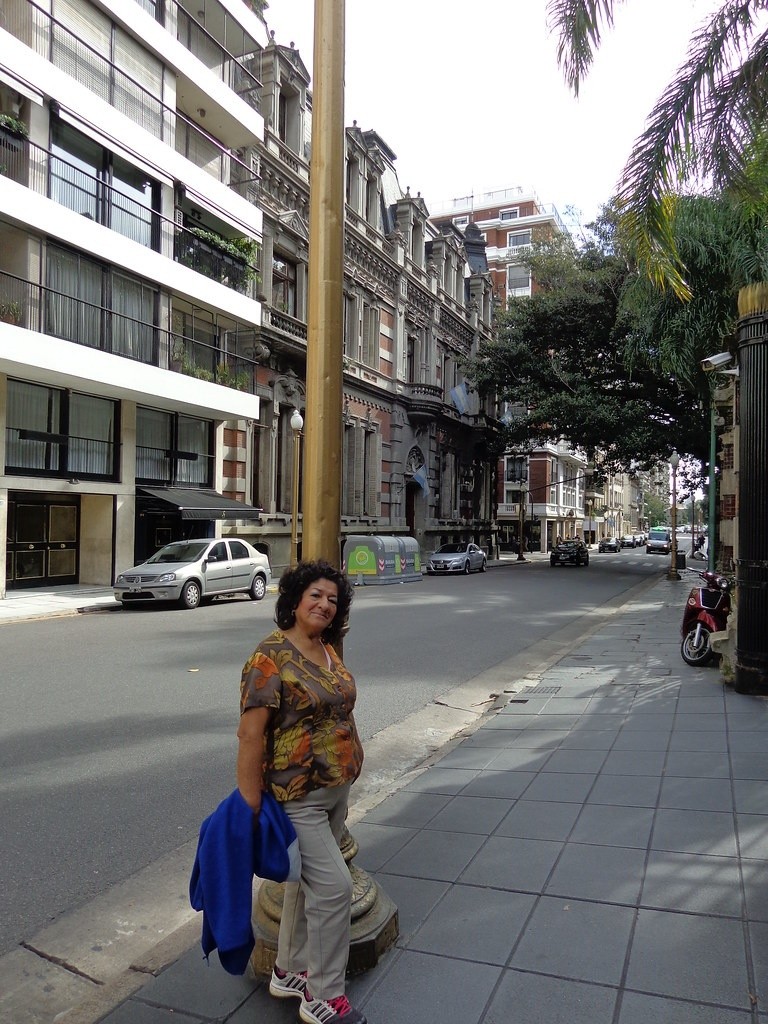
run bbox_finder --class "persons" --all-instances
[556,534,562,544]
[607,532,611,537]
[566,534,570,540]
[698,534,705,550]
[523,535,529,551]
[237,560,365,1024]
[574,534,578,538]
[511,535,517,554]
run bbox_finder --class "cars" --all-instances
[619,531,649,549]
[598,537,621,553]
[426,543,487,575]
[669,525,709,533]
[113,538,271,609]
[670,537,679,551]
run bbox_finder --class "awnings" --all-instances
[137,486,263,519]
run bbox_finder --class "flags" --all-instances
[450,384,471,415]
[414,464,430,499]
[501,412,515,431]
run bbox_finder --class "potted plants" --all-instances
[0,300,24,325]
[177,226,264,291]
[172,345,250,393]
[0,113,31,153]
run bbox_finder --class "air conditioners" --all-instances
[174,209,184,234]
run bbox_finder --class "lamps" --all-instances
[67,479,80,485]
[191,208,203,220]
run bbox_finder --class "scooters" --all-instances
[681,567,734,667]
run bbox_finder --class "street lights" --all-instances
[617,505,651,540]
[291,410,303,572]
[587,500,593,549]
[668,450,682,581]
[517,483,527,559]
[691,494,703,558]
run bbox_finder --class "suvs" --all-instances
[550,540,589,568]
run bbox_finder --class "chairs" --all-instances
[209,547,218,556]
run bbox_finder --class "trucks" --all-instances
[646,527,671,554]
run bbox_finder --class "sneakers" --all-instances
[270,963,308,999]
[298,982,367,1024]
